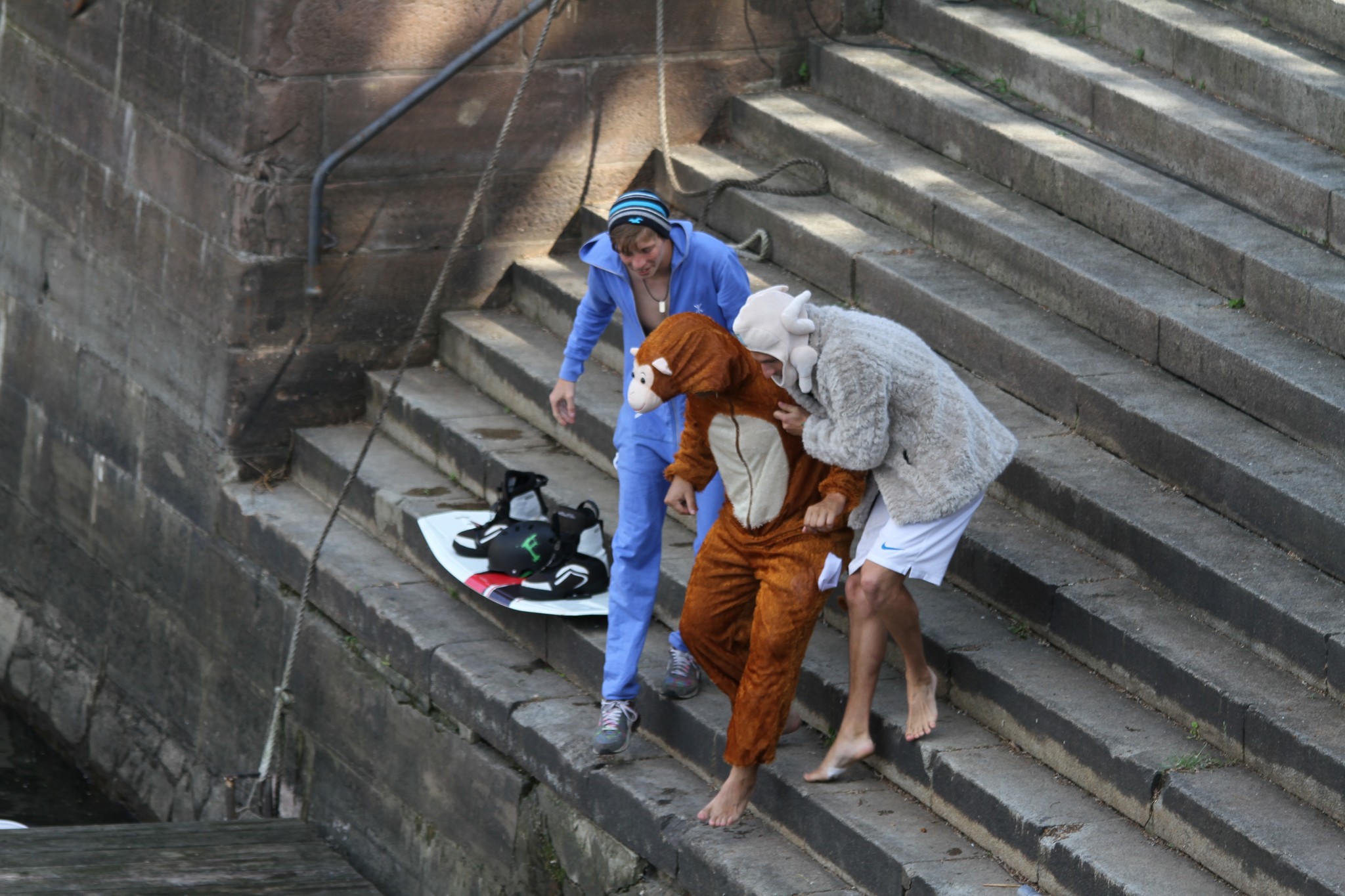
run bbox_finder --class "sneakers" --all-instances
[593,698,637,755]
[661,645,702,700]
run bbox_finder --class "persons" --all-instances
[549,187,1019,827]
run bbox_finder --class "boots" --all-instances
[521,500,610,601]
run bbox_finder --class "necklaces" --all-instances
[642,268,670,313]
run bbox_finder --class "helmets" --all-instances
[488,521,556,578]
[453,470,548,558]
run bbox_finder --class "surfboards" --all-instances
[417,509,610,616]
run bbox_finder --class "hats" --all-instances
[607,188,672,239]
